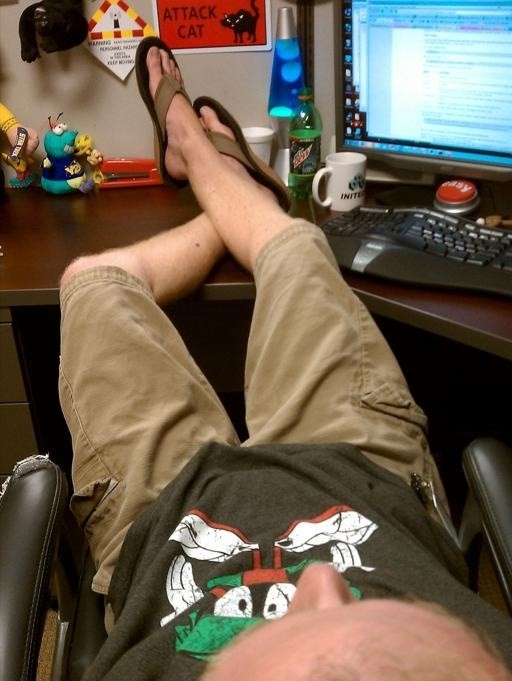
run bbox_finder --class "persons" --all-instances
[54,36,511,681]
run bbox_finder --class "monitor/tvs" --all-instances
[334,0,512,184]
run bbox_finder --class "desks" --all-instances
[0,179,512,491]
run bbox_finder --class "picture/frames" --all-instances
[151,0,272,54]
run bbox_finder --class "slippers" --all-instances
[190,90,290,215]
[134,34,195,188]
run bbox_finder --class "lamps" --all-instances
[266,6,307,186]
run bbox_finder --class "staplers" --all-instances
[97,158,165,190]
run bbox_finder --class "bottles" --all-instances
[285,83,323,200]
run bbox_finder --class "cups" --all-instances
[312,149,370,215]
[240,125,276,168]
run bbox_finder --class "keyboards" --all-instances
[320,205,512,295]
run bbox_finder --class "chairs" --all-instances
[0,437,512,681]
